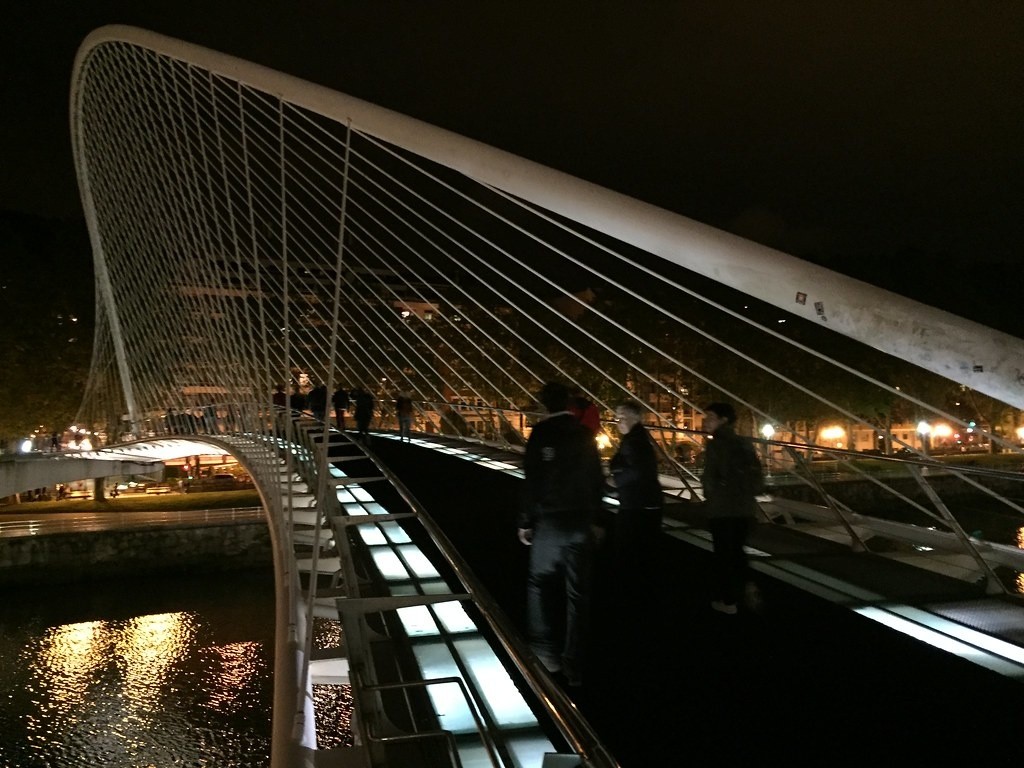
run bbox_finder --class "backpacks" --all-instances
[712,435,762,496]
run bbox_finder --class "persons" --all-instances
[51,430,60,448]
[273,381,414,446]
[113,482,118,498]
[703,406,763,613]
[608,403,667,594]
[26,486,66,502]
[164,401,248,434]
[519,381,606,674]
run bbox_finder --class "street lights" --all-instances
[917,421,931,471]
[760,423,775,478]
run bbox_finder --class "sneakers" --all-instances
[525,645,561,673]
[562,659,584,687]
[744,582,759,603]
[710,600,738,615]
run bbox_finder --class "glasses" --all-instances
[613,417,636,423]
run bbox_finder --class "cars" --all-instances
[206,474,237,481]
